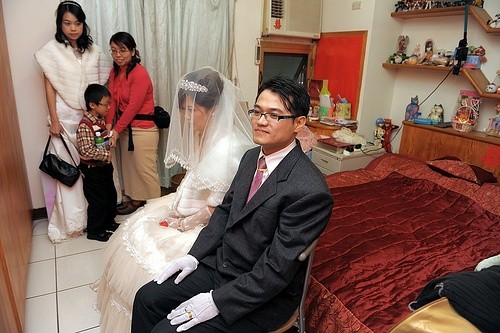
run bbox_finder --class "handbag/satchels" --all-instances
[39,133,80,187]
[116,106,171,129]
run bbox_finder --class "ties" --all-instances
[246,157,267,204]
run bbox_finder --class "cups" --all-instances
[335,102,352,119]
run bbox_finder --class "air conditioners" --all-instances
[262,0,322,38]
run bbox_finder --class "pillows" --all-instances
[425,155,498,186]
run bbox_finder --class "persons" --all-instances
[104,32,161,215]
[374,119,399,152]
[130,77,334,333]
[76,84,121,242]
[97,66,260,333]
[34,0,112,244]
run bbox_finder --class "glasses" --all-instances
[247,109,307,121]
[109,49,130,53]
[99,102,112,107]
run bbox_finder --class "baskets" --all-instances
[451,105,477,132]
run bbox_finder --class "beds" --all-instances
[304,121,500,333]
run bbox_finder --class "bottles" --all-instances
[307,79,335,121]
[95,132,106,150]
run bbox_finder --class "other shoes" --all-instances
[107,223,120,231]
[116,201,129,208]
[87,232,113,241]
[84,228,89,232]
[118,200,146,215]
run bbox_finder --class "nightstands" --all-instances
[311,145,385,176]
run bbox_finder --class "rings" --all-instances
[185,307,187,312]
[188,312,192,318]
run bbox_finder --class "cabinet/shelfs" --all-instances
[306,121,358,138]
[382,5,500,99]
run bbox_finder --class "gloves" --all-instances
[167,290,220,332]
[153,254,199,284]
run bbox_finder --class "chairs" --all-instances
[269,237,320,333]
